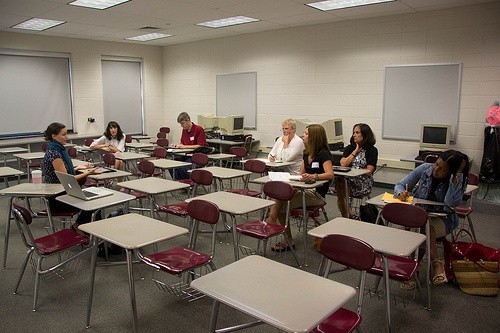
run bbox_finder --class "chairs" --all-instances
[11,137,500,332]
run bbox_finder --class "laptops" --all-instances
[54,171,112,200]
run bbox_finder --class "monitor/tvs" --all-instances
[197,114,218,129]
[321,118,344,144]
[420,123,451,148]
[216,115,244,133]
[295,118,315,138]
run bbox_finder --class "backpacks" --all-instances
[97,207,132,256]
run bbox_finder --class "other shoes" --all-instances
[70,223,85,236]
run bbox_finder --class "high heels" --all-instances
[432,258,448,284]
[400,269,420,290]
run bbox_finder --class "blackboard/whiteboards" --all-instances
[216,72,257,130]
[382,63,461,143]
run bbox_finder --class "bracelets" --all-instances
[314,174,320,181]
[350,153,356,158]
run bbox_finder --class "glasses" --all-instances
[280,127,293,132]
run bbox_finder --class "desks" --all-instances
[0,141,479,333]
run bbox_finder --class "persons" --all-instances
[166,112,209,194]
[336,124,378,220]
[90,121,126,191]
[394,150,470,289]
[42,123,98,239]
[266,119,304,218]
[265,125,334,251]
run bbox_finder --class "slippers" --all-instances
[271,242,295,251]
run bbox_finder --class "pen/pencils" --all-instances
[406,184,408,191]
[270,154,274,158]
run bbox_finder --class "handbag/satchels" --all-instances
[358,204,386,227]
[443,230,500,298]
